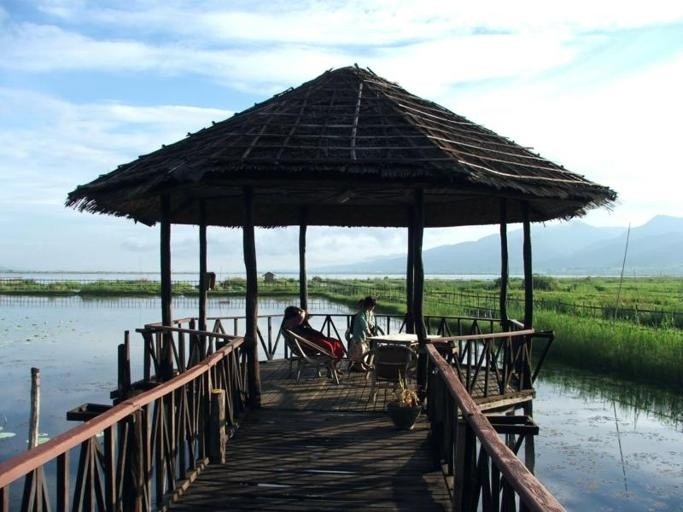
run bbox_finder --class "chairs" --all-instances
[281,324,417,392]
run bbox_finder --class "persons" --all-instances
[280,306,344,380]
[347,295,379,372]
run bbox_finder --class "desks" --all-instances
[366,332,443,378]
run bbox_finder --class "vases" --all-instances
[387,368,423,428]
